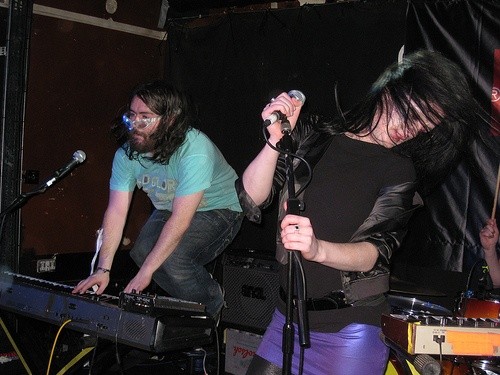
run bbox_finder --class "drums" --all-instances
[435,356,472,375]
[457,290,500,320]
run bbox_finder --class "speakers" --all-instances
[222,246,286,334]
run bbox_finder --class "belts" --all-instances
[279,284,350,311]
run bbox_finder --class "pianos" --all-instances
[0,271,221,375]
[380,312,500,357]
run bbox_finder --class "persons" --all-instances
[234,49,477,375]
[479,217,500,288]
[71,80,245,326]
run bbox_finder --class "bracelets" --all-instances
[96,267,110,273]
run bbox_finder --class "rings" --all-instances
[269,103,271,107]
[294,225,300,235]
[270,97,275,101]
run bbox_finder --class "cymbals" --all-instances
[388,293,454,316]
[389,275,447,296]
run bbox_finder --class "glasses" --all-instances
[122,112,162,131]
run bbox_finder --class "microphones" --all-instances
[46,150,86,187]
[264,90,305,127]
